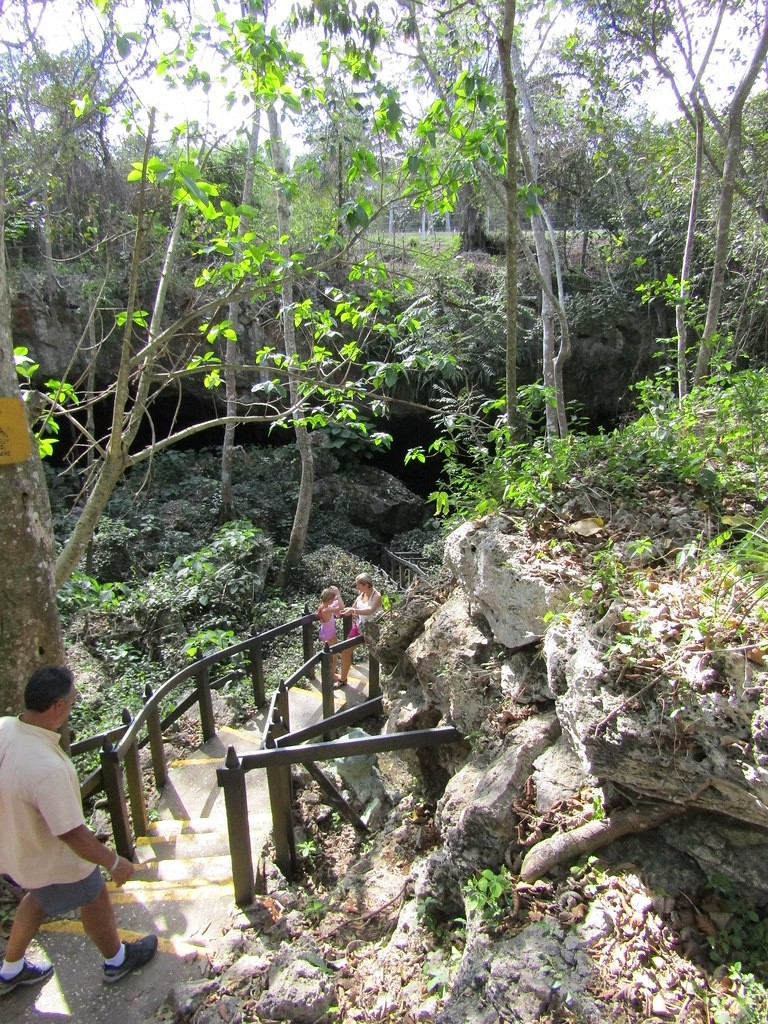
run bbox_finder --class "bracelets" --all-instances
[337,595,340,596]
[352,609,355,615]
[109,855,119,873]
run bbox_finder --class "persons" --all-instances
[319,585,345,682]
[0,663,159,997]
[333,573,382,689]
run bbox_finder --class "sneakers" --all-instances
[0,958,54,994]
[103,934,158,983]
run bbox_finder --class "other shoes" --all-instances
[332,678,347,689]
[331,674,341,681]
